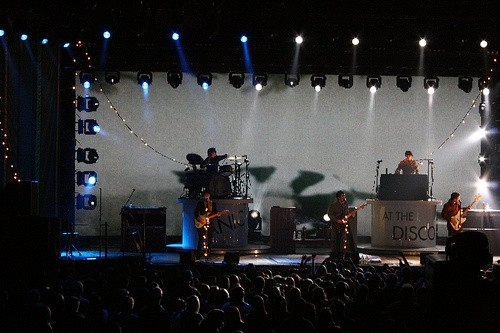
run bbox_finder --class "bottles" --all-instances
[361,256,368,265]
[129,200,132,208]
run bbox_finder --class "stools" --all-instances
[61,232,82,256]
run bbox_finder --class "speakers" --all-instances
[270,206,295,255]
[121,206,167,252]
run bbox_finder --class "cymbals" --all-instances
[186,153,203,165]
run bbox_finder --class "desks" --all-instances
[121,208,166,253]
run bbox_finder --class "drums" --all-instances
[207,175,231,198]
[219,165,232,176]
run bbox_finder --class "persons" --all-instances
[8,252,500,333]
[441,193,471,239]
[394,151,420,175]
[203,147,230,177]
[194,190,221,261]
[328,190,360,256]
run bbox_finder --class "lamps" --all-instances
[0,25,85,49]
[75,119,100,135]
[167,25,181,40]
[287,32,305,49]
[79,68,473,93]
[101,24,112,42]
[75,171,97,186]
[234,31,250,44]
[74,95,100,113]
[75,147,99,164]
[75,193,97,210]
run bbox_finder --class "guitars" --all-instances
[450,192,483,230]
[332,202,368,231]
[195,209,230,228]
[125,218,142,252]
